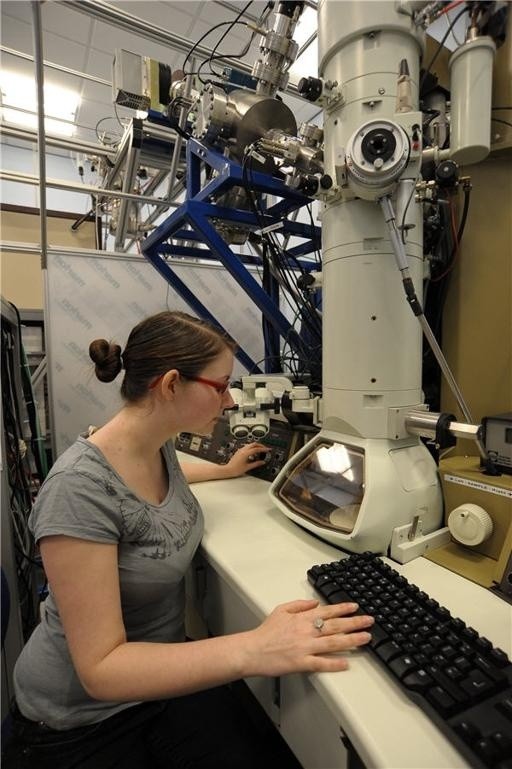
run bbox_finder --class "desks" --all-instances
[174,448,512,769]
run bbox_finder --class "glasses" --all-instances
[148,371,230,395]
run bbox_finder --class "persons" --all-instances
[0,308,376,769]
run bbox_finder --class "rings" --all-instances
[314,618,324,629]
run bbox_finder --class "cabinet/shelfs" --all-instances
[16,309,54,449]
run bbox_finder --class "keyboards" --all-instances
[306,548,511,768]
